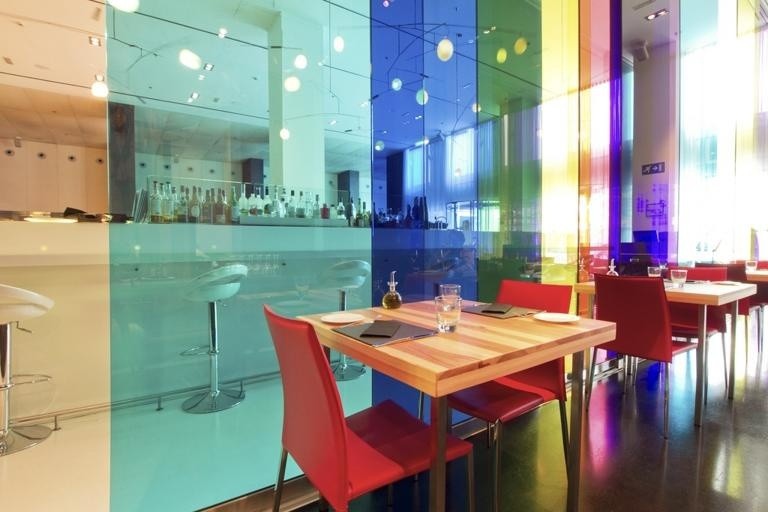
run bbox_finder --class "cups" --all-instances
[435,295,461,332]
[669,269,688,288]
[745,258,757,273]
[647,266,662,278]
[439,284,459,299]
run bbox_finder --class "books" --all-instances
[331,319,437,347]
[461,303,541,320]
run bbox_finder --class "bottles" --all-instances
[149,179,369,227]
[374,195,427,228]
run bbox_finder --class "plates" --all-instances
[533,312,579,323]
[320,311,363,324]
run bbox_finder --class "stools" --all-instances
[1,283,59,457]
[169,260,249,417]
